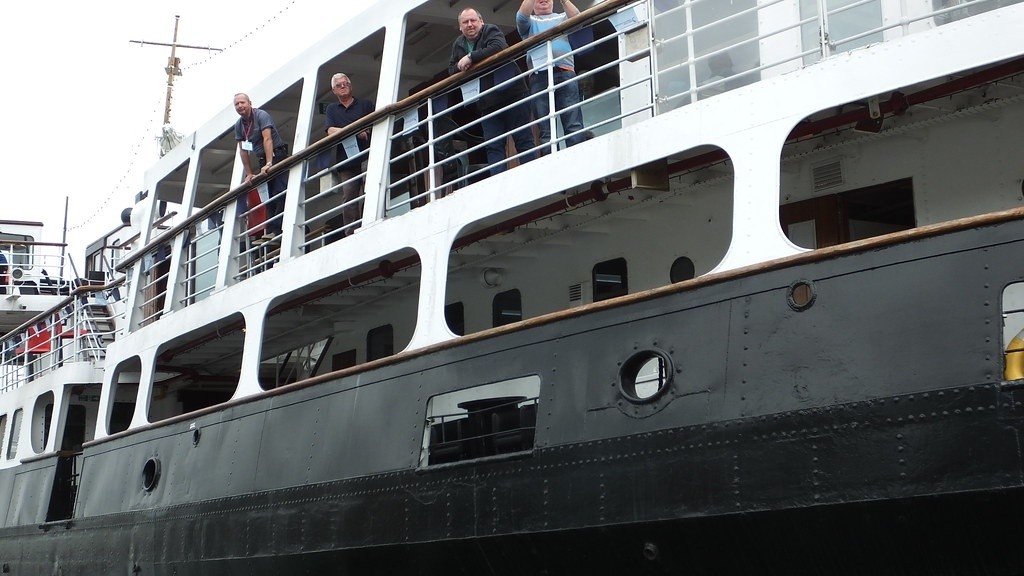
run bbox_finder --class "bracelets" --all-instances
[467,52,472,61]
[560,0,569,7]
[266,162,272,167]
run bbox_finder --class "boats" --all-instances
[2,0,1024,576]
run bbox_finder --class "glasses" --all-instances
[336,81,350,87]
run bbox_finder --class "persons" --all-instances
[233,91,289,246]
[448,6,538,177]
[707,52,742,92]
[324,71,375,237]
[0,250,8,294]
[516,0,595,157]
